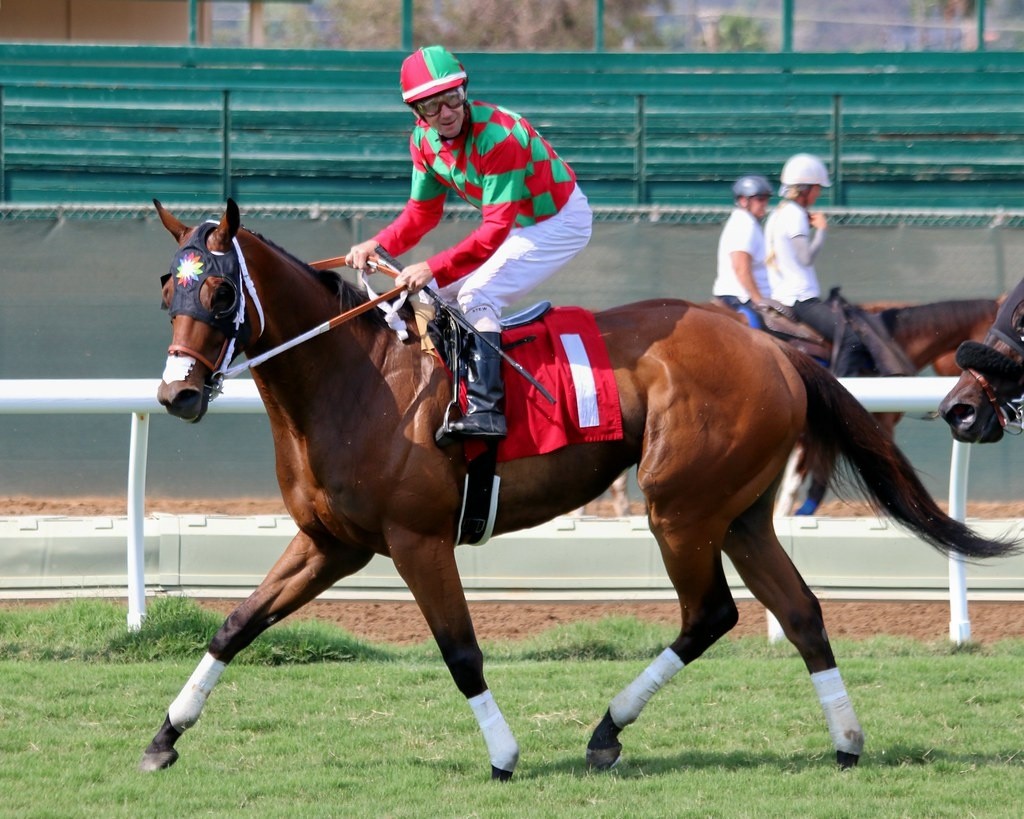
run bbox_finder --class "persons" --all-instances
[345,45,592,441]
[712,177,773,329]
[765,155,863,376]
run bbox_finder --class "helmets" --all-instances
[733,176,773,199]
[780,153,832,187]
[400,45,469,104]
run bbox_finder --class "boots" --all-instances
[434,332,507,445]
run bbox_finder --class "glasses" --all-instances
[414,85,466,116]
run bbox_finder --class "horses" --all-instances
[135,197,1024,780]
